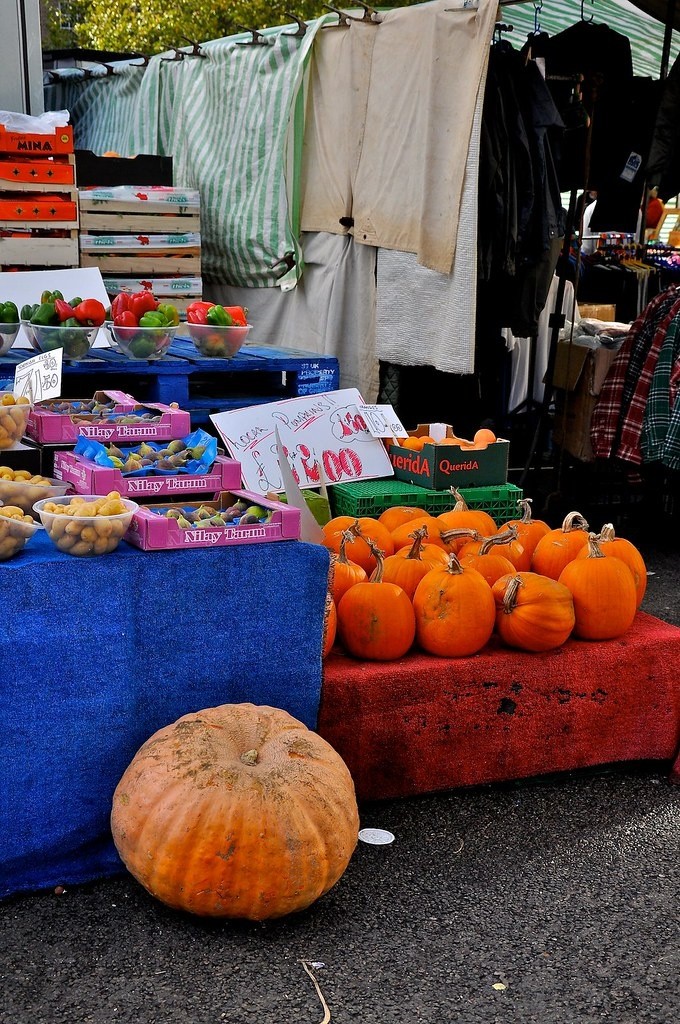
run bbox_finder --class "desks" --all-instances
[0,346,340,424]
[0,530,333,902]
[308,610,680,800]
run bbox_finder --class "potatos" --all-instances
[0,465,134,559]
[0,394,31,449]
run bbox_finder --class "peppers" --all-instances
[0,300,19,335]
[186,301,248,357]
[106,290,179,358]
[20,290,106,358]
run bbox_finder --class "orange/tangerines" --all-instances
[384,428,495,453]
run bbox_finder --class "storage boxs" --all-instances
[0,123,203,315]
[15,390,302,551]
[541,338,619,462]
[382,424,510,491]
[328,477,523,530]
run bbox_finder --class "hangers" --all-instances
[559,232,680,279]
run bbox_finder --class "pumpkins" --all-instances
[320,486,646,661]
[110,703,361,921]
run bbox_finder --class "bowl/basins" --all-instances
[0,320,181,359]
[0,514,44,562]
[0,475,71,519]
[32,494,141,557]
[185,320,254,359]
[0,403,34,450]
